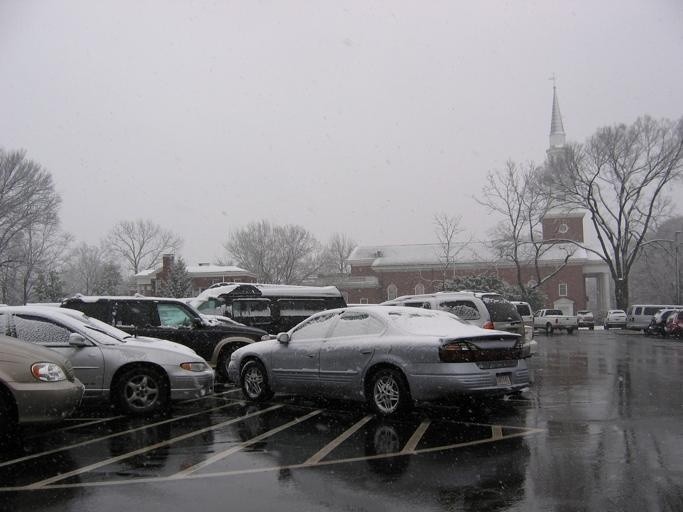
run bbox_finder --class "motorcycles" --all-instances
[644,316,682,337]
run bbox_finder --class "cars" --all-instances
[0,301,215,419]
[227,304,533,420]
[231,399,533,512]
[0,333,85,438]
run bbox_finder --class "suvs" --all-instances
[662,310,682,331]
[58,292,269,385]
[602,309,627,330]
[377,289,532,359]
[654,309,682,323]
[574,310,596,330]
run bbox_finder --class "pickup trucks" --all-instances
[531,308,579,334]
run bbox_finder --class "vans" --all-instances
[170,281,347,335]
[510,300,537,355]
[627,305,682,332]
